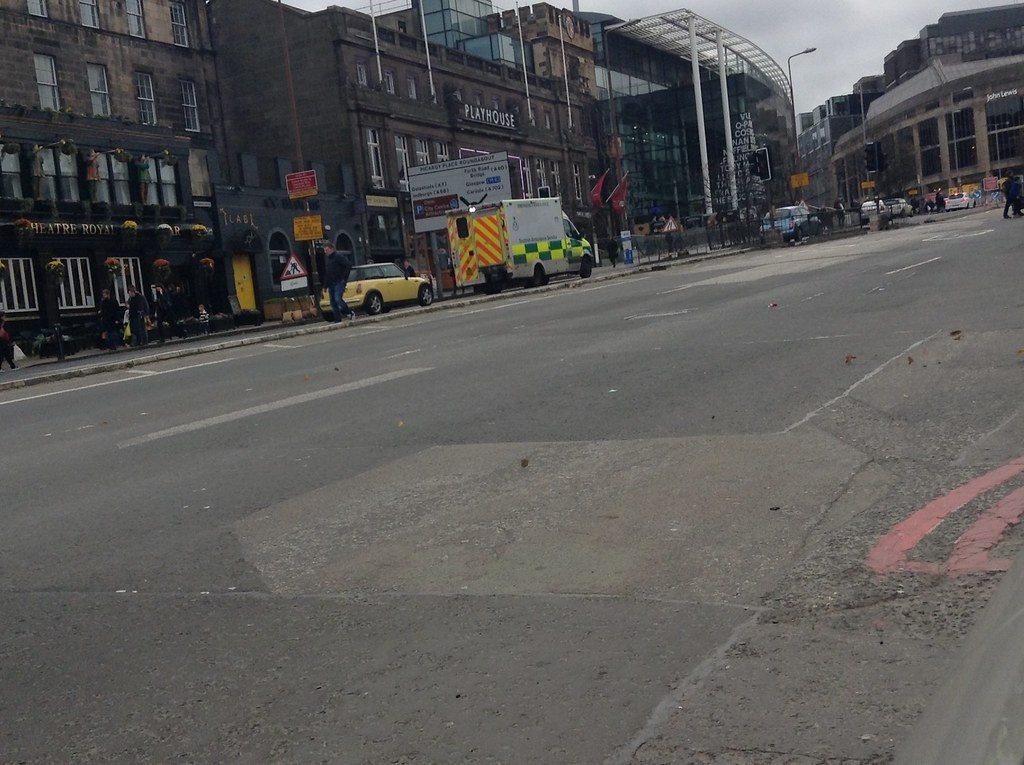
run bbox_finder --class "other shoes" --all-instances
[348,312,355,320]
[1004,216,1010,219]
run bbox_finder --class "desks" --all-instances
[160,326,180,342]
[186,321,201,336]
[212,318,235,331]
[235,313,264,328]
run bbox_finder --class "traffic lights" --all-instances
[755,147,771,181]
[746,151,759,175]
[864,143,878,173]
[877,142,889,172]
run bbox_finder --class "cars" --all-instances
[885,198,914,218]
[860,200,891,224]
[319,262,433,322]
[944,192,976,212]
[759,206,823,242]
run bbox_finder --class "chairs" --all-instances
[62,323,100,352]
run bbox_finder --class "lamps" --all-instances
[21,137,78,200]
[134,149,177,206]
[0,143,21,161]
[87,148,133,201]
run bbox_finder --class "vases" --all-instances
[159,234,170,241]
[127,232,135,237]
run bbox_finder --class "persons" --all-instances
[101,282,188,352]
[323,243,355,323]
[835,194,845,227]
[651,215,666,233]
[199,305,211,336]
[450,258,464,296]
[910,191,947,214]
[607,235,618,268]
[1003,171,1024,219]
[0,311,19,373]
[404,260,416,277]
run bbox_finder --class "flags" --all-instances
[591,170,627,213]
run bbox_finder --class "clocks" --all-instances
[566,15,575,40]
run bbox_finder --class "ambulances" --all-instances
[445,197,594,294]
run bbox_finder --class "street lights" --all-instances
[788,48,816,201]
[950,86,972,193]
[985,122,1009,189]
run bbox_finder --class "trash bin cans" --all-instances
[478,263,506,296]
[869,213,890,231]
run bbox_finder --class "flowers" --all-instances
[0,262,5,270]
[122,220,137,233]
[47,261,65,278]
[155,223,174,234]
[191,224,207,239]
[15,218,33,240]
[200,258,215,269]
[104,258,129,277]
[150,258,170,269]
